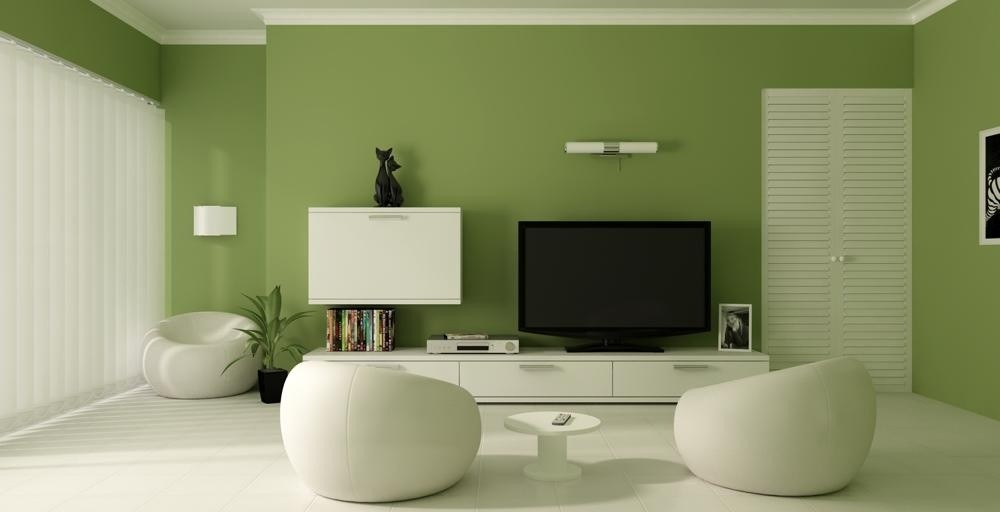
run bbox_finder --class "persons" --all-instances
[722,308,750,349]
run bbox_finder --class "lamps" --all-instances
[193,205,237,237]
[562,141,659,158]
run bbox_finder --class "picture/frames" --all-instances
[977,126,1000,246]
[718,303,752,353]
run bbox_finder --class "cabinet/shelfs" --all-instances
[307,206,463,306]
[760,86,915,394]
[303,345,770,404]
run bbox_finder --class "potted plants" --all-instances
[221,284,317,403]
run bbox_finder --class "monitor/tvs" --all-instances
[516,218,712,352]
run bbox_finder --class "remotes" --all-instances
[552,413,572,425]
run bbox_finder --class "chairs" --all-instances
[673,356,877,498]
[136,310,266,399]
[279,360,482,503]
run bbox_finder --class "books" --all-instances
[444,331,489,339]
[325,307,396,352]
